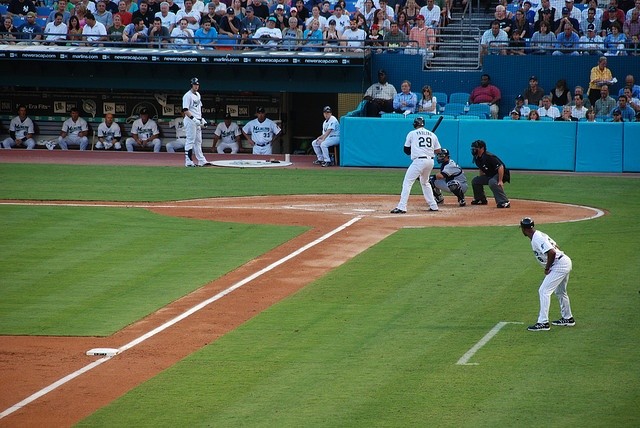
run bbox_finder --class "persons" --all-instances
[123,17,148,42]
[550,80,572,106]
[269,0,291,19]
[201,3,221,35]
[419,0,440,42]
[530,21,556,55]
[533,8,556,34]
[295,0,309,23]
[567,86,592,109]
[396,12,410,35]
[363,70,398,117]
[601,5,623,36]
[618,74,640,100]
[402,0,420,29]
[433,0,447,42]
[107,15,125,42]
[50,0,75,13]
[395,0,422,15]
[216,7,241,51]
[46,0,70,27]
[0,17,18,45]
[393,80,417,114]
[237,28,259,51]
[554,7,579,39]
[610,95,635,121]
[324,4,351,35]
[194,18,218,50]
[451,0,468,13]
[75,0,96,19]
[579,23,603,56]
[3,105,36,150]
[147,17,170,48]
[604,22,628,57]
[446,0,453,24]
[319,1,333,19]
[133,0,142,10]
[302,19,323,51]
[494,5,513,42]
[284,7,303,32]
[232,0,252,9]
[146,0,158,14]
[82,13,108,47]
[623,9,640,39]
[93,113,122,151]
[312,106,340,167]
[373,0,395,23]
[121,0,138,14]
[602,0,625,27]
[580,0,597,5]
[241,6,263,36]
[7,0,37,18]
[267,4,290,32]
[552,23,580,56]
[586,109,596,122]
[317,0,327,11]
[626,0,640,20]
[158,0,180,14]
[211,113,244,154]
[511,110,520,120]
[182,77,211,167]
[580,7,603,37]
[610,107,624,122]
[510,10,530,38]
[247,0,269,27]
[418,85,437,115]
[509,94,530,117]
[570,94,589,120]
[471,140,510,208]
[282,17,303,51]
[537,95,561,118]
[468,74,501,119]
[360,0,378,31]
[154,2,176,34]
[522,0,539,12]
[175,0,201,32]
[617,86,640,118]
[16,12,42,45]
[101,0,119,15]
[373,9,390,40]
[581,0,603,22]
[93,2,113,31]
[191,0,204,14]
[527,110,540,120]
[340,19,367,52]
[170,19,194,49]
[115,1,132,26]
[251,17,282,51]
[158,109,187,152]
[477,20,508,70]
[627,34,640,56]
[356,13,368,33]
[511,1,535,38]
[32,0,45,7]
[538,0,555,21]
[390,117,442,213]
[408,14,435,70]
[429,148,468,207]
[520,218,576,331]
[40,12,68,46]
[125,108,162,152]
[353,0,378,20]
[587,56,617,108]
[203,0,227,17]
[305,5,327,34]
[415,0,428,11]
[332,0,351,16]
[595,85,616,115]
[132,1,155,29]
[554,105,578,121]
[58,107,89,150]
[71,2,89,30]
[499,0,512,19]
[364,23,384,57]
[560,0,581,23]
[524,76,545,106]
[242,107,282,155]
[383,22,408,54]
[233,0,246,20]
[323,20,343,52]
[66,15,83,46]
[509,29,526,56]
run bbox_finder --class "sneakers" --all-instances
[435,197,444,203]
[390,207,407,213]
[528,323,550,330]
[460,199,466,206]
[313,160,323,164]
[322,161,331,167]
[497,201,510,208]
[471,199,487,205]
[185,161,201,167]
[552,317,575,326]
[198,161,213,166]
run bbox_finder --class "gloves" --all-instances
[202,118,209,129]
[192,117,201,126]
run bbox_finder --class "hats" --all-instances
[226,7,235,13]
[563,6,570,13]
[329,19,336,25]
[415,15,424,20]
[613,108,621,114]
[515,94,524,100]
[608,6,617,12]
[290,7,297,12]
[267,16,277,22]
[371,24,379,29]
[587,23,596,31]
[528,75,537,80]
[512,28,521,34]
[378,67,386,74]
[323,106,332,112]
[511,107,520,114]
[208,2,216,8]
[245,6,253,11]
[140,108,149,114]
[277,4,284,9]
[542,8,550,14]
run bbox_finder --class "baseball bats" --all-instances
[431,116,443,133]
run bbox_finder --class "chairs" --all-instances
[384,115,401,119]
[450,93,468,103]
[433,92,447,103]
[441,102,464,114]
[433,114,454,121]
[469,104,489,114]
[408,114,428,118]
[457,114,485,120]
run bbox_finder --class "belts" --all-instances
[418,156,435,159]
[558,253,564,259]
[254,142,269,147]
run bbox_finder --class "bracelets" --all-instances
[147,139,149,142]
[190,116,193,119]
[21,137,27,142]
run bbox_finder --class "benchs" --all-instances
[0,120,254,152]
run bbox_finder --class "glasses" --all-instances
[516,13,523,15]
[350,20,357,22]
[423,89,429,92]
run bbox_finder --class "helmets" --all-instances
[437,148,449,163]
[190,77,200,84]
[521,218,534,228]
[471,140,486,163]
[414,117,425,128]
